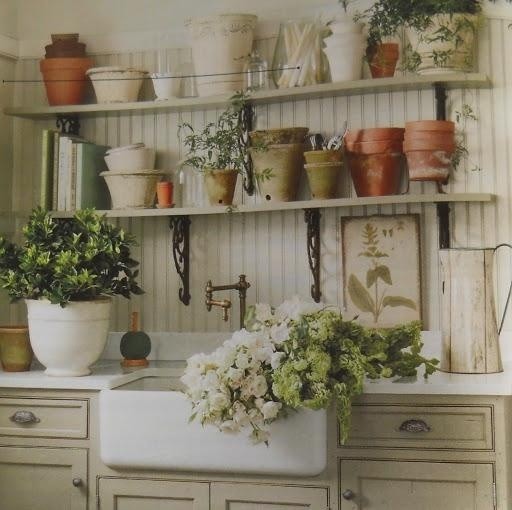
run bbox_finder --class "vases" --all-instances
[32,30,95,105]
[301,143,344,199]
[247,125,308,200]
[344,122,405,197]
[97,140,164,211]
[401,117,458,183]
[152,180,177,206]
[81,65,148,105]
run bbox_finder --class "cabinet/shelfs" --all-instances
[305,363,512,496]
[3,68,498,309]
[0,358,115,510]
[92,468,338,510]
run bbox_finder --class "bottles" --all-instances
[242,49,267,94]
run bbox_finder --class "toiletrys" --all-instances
[119,312,151,367]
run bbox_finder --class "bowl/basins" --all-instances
[86,66,149,104]
[247,127,309,203]
[341,126,406,197]
[404,120,457,184]
[38,57,96,106]
[98,142,166,209]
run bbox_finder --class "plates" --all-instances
[154,203,176,209]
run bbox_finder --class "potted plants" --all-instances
[348,0,511,79]
[170,100,255,208]
[1,195,151,378]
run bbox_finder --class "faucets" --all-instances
[205,275,251,330]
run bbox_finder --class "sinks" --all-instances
[98,368,328,477]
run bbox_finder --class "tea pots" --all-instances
[434,240,512,375]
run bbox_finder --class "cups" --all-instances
[302,149,344,199]
[150,71,184,100]
[156,181,174,205]
[322,24,369,84]
[365,41,399,78]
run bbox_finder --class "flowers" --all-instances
[173,292,447,448]
